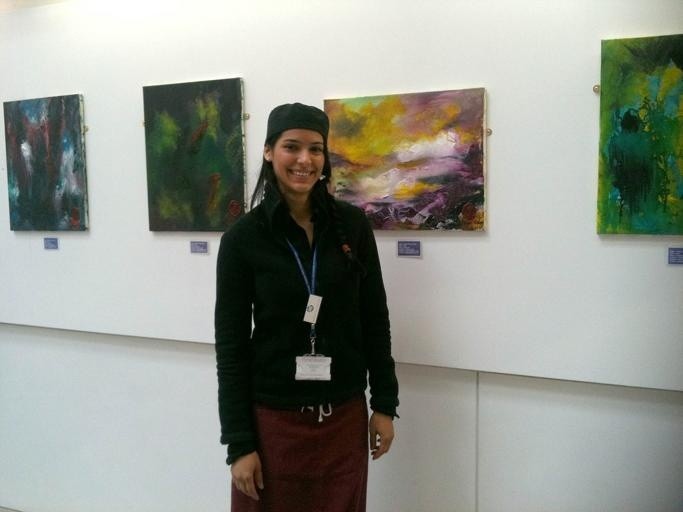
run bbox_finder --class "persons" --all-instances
[211,102,401,511]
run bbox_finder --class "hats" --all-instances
[267,103,330,142]
